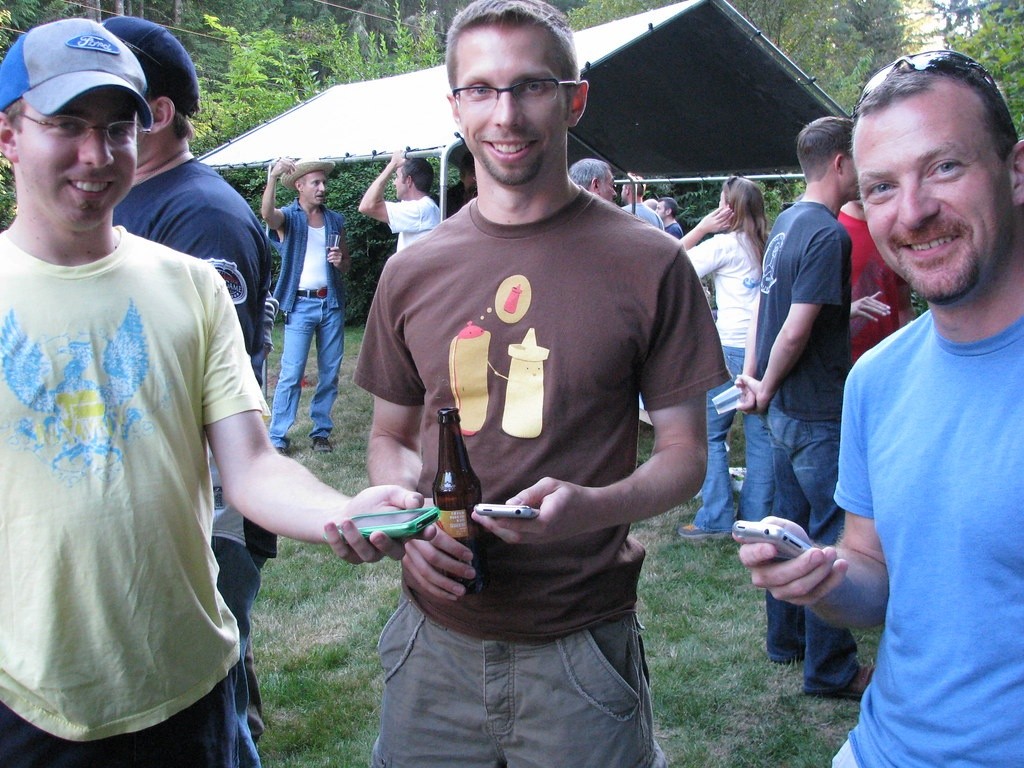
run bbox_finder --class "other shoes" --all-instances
[805,666,875,698]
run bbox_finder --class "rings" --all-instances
[719,218,723,222]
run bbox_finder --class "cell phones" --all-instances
[732,521,813,562]
[474,503,540,519]
[323,507,441,542]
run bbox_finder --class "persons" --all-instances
[677,175,775,541]
[353,0,733,768]
[735,117,914,700]
[0,18,436,768]
[732,50,1024,768]
[568,158,684,241]
[359,149,479,253]
[261,154,350,456]
[104,18,277,749]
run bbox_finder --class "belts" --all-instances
[296,287,327,299]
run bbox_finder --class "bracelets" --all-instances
[270,173,280,182]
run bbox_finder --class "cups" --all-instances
[712,385,746,415]
[326,234,340,254]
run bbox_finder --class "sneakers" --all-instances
[309,436,332,452]
[677,524,732,542]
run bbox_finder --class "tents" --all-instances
[200,0,848,400]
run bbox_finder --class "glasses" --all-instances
[452,78,576,110]
[851,50,999,118]
[16,111,151,145]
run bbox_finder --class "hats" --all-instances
[102,15,199,118]
[0,18,154,128]
[281,157,335,189]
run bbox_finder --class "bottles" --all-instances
[433,408,487,596]
